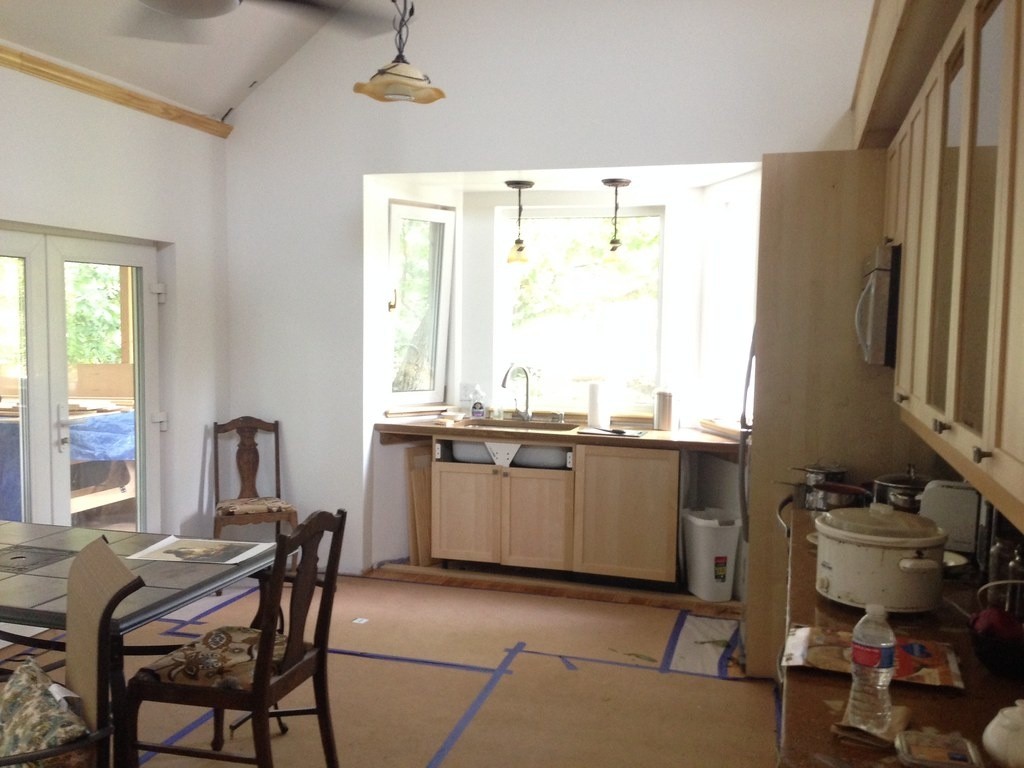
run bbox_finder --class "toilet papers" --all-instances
[588,381,610,430]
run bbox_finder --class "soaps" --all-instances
[434,418,453,425]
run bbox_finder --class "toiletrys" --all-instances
[470,384,484,419]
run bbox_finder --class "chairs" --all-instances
[213,415,298,596]
[128,507,348,768]
[0,534,145,767]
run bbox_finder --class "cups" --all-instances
[492,406,504,420]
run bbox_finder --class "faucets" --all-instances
[501,363,533,420]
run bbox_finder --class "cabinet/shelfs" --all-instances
[431,430,681,583]
[880,1,1024,536]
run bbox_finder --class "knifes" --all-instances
[595,427,625,433]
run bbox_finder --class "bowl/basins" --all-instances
[983,699,1024,768]
[441,412,465,421]
[893,731,982,768]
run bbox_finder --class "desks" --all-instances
[0,519,289,768]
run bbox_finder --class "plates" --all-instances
[943,551,968,566]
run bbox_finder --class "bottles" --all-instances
[848,603,897,733]
[987,538,1015,605]
[1005,544,1024,622]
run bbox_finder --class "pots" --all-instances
[815,503,949,613]
[787,455,852,485]
[771,479,872,511]
[873,464,933,515]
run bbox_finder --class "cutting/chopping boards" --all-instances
[577,426,648,437]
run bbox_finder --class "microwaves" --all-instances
[854,245,902,370]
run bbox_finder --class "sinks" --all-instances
[452,419,579,467]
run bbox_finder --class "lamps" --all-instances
[352,0,447,104]
[140,0,243,20]
[601,179,632,261]
[505,181,535,263]
